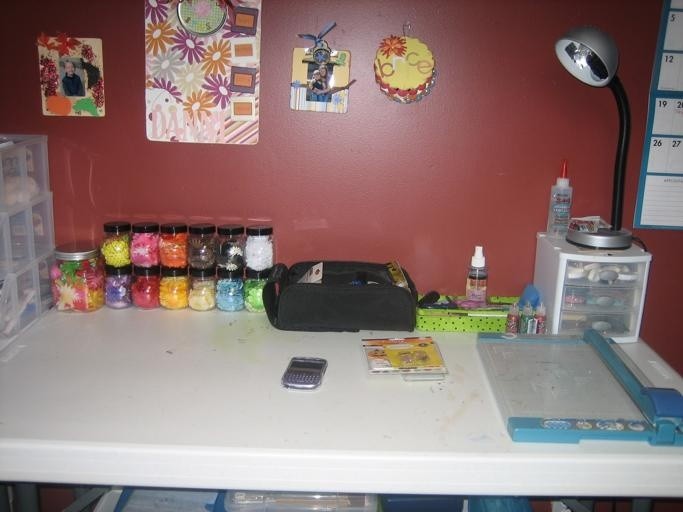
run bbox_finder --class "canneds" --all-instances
[133,267,161,310]
[97,222,130,269]
[187,223,219,268]
[243,274,270,312]
[102,265,133,308]
[188,269,217,311]
[159,224,187,268]
[216,225,245,271]
[127,221,159,269]
[216,272,244,312]
[158,269,190,309]
[246,226,274,274]
[51,241,107,312]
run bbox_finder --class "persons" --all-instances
[61,59,85,96]
[309,70,326,102]
[308,65,331,102]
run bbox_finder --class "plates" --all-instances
[178,0,228,38]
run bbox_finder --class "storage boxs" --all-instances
[0,133,55,355]
[533,232,653,343]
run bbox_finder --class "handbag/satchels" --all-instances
[262,261,418,332]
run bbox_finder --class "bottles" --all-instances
[504,302,519,334]
[533,302,546,335]
[47,217,276,314]
[546,159,574,240]
[518,301,532,335]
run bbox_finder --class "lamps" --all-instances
[555,26,634,249]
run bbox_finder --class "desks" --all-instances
[0,310,683,511]
